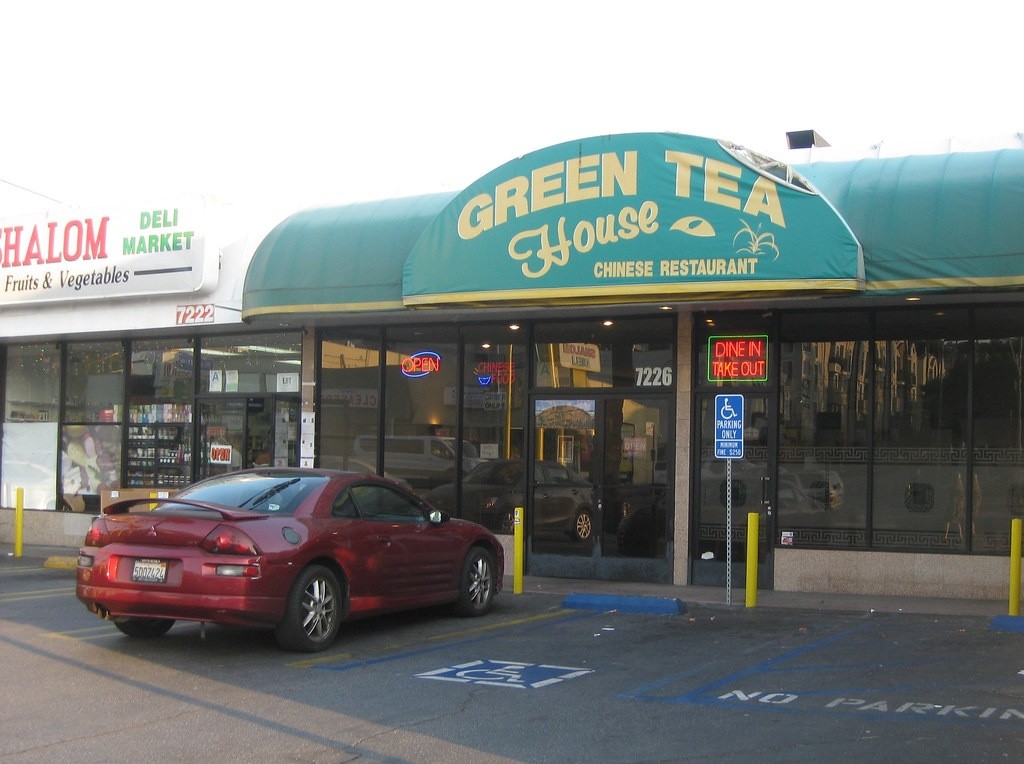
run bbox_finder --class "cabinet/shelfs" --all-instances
[128,422,207,488]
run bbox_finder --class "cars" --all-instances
[805,481,835,502]
[422,459,598,543]
[320,456,414,510]
[76,467,505,653]
[700,474,827,527]
[2,460,85,512]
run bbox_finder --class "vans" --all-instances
[353,435,489,482]
